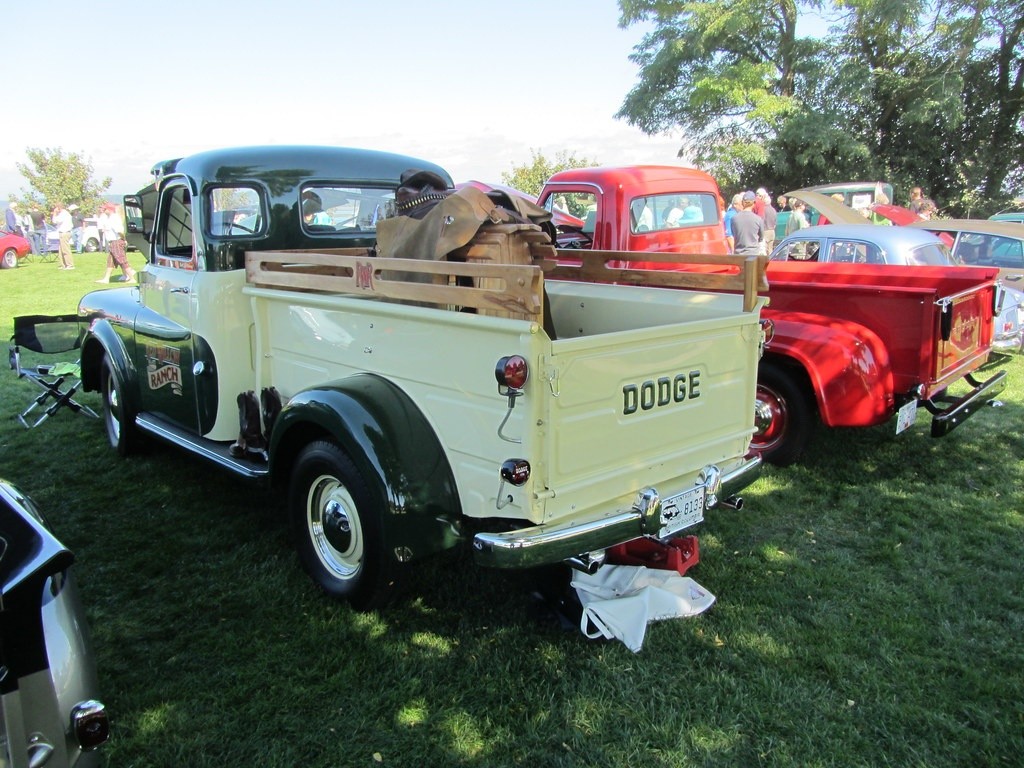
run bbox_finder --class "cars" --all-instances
[0,230,32,270]
[44,217,138,253]
[768,181,1024,340]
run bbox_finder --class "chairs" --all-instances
[958,242,995,267]
[635,209,685,231]
[19,239,61,262]
[7,314,99,430]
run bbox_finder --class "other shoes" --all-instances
[119,269,137,280]
[95,279,109,284]
[58,265,75,270]
[127,278,137,283]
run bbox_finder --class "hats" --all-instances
[99,203,115,212]
[69,204,79,211]
[795,200,802,207]
[677,206,703,223]
[743,191,755,201]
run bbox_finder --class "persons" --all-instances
[860,193,891,226]
[52,202,75,270]
[5,202,48,256]
[774,196,791,212]
[817,193,844,225]
[785,200,810,235]
[662,195,704,227]
[788,198,798,210]
[94,202,137,283]
[910,187,936,221]
[553,196,569,214]
[303,212,333,225]
[720,186,776,256]
[69,205,85,254]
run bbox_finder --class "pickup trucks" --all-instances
[453,162,1010,463]
[78,143,780,613]
